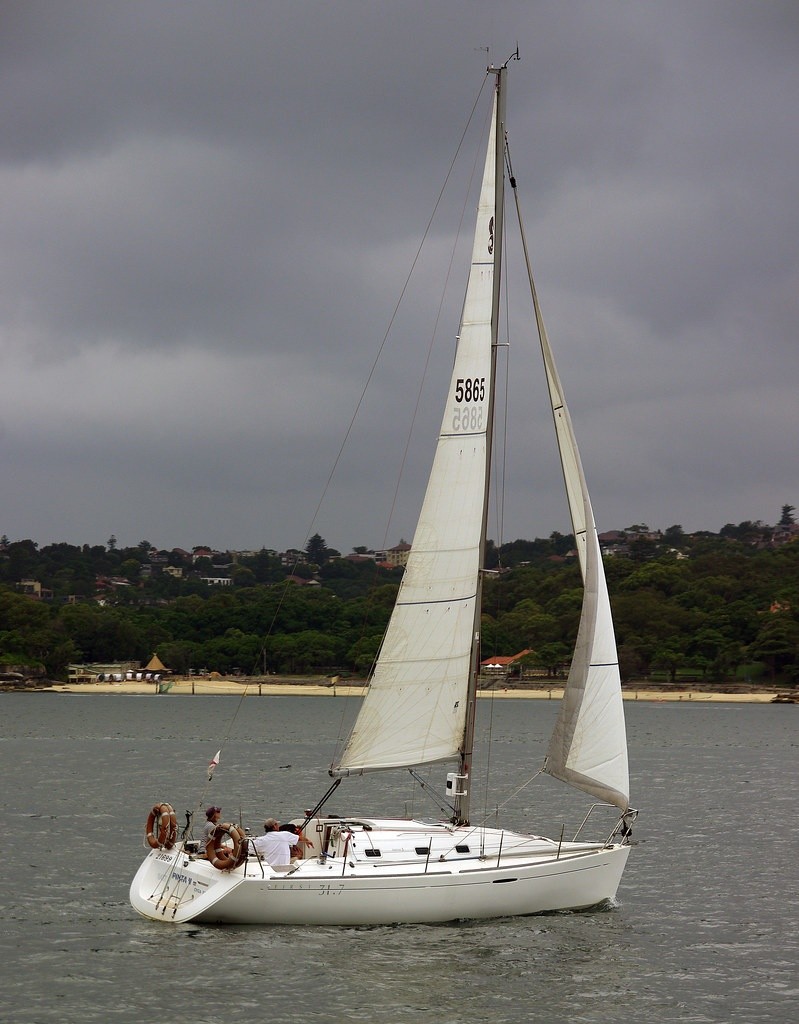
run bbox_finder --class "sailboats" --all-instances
[126,51,639,926]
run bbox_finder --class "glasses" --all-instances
[216,811,220,813]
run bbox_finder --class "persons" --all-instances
[248,817,315,867]
[199,806,232,854]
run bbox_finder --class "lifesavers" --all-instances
[145,801,178,851]
[205,822,248,870]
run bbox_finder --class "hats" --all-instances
[205,807,221,820]
[264,818,280,829]
